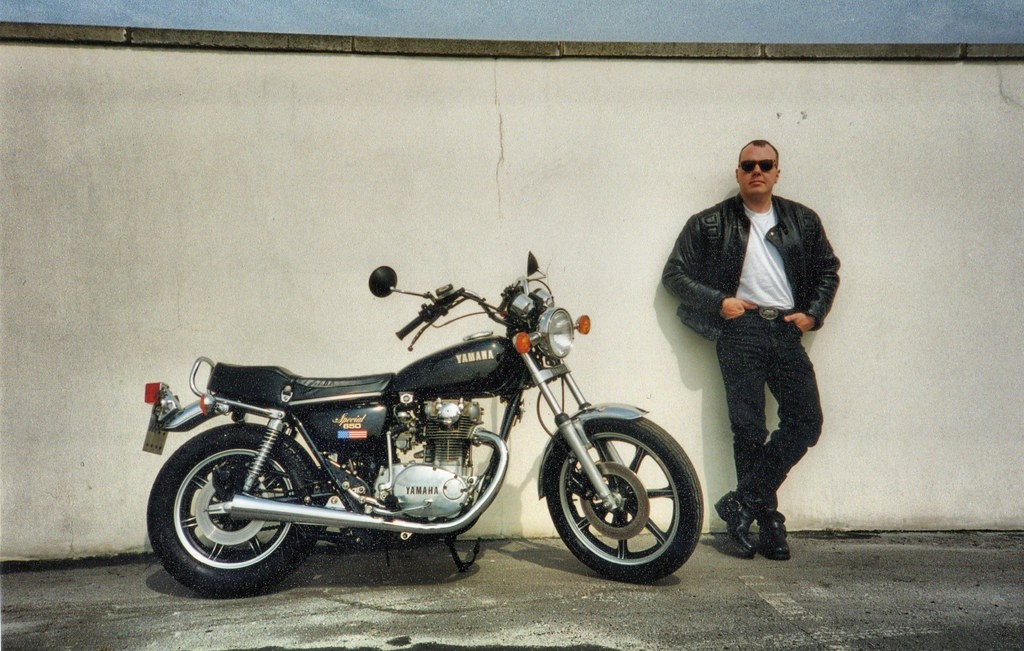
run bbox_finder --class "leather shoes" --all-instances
[758,512,789,560]
[715,491,756,556]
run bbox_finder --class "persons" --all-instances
[660,138,840,559]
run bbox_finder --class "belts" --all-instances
[744,307,796,320]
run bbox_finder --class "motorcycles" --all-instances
[141,251,705,601]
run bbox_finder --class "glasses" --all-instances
[738,160,777,173]
